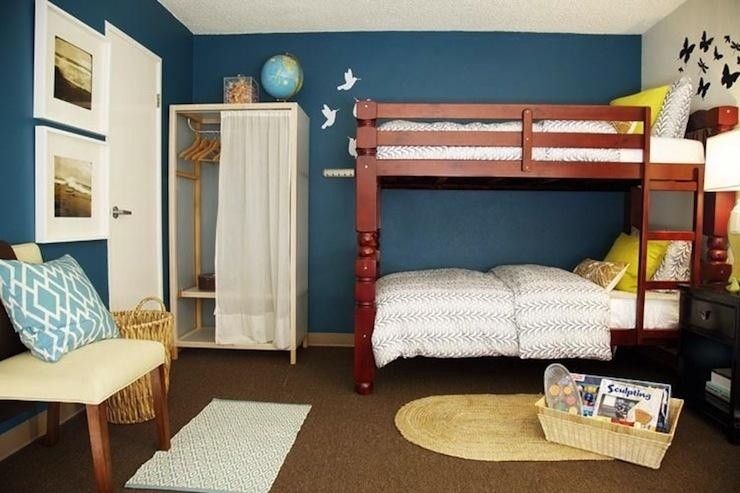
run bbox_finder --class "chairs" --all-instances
[0,240,172,492]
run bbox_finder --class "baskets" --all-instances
[533,393,686,471]
[105,296,175,423]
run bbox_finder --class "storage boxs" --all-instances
[224,77,260,103]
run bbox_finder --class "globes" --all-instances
[260,52,304,102]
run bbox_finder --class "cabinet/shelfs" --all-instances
[167,102,311,365]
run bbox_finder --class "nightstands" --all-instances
[674,284,740,447]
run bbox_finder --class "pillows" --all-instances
[650,75,694,138]
[602,233,670,293]
[0,254,121,363]
[630,226,693,293]
[573,258,630,292]
[609,85,672,135]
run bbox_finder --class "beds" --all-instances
[354,100,740,397]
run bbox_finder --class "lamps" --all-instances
[702,128,740,293]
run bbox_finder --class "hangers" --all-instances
[177,130,220,164]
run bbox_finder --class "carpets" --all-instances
[395,394,615,461]
[123,398,312,493]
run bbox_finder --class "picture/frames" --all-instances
[34,126,112,244]
[33,1,110,137]
